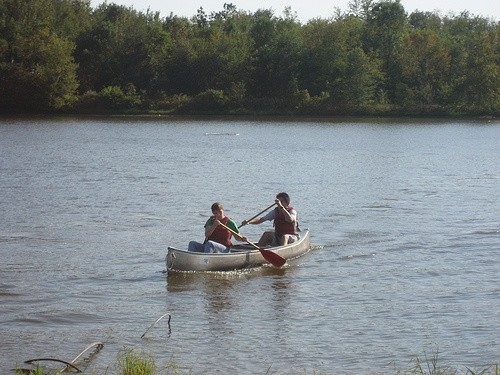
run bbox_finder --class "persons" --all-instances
[188,203,248,256]
[243,192,298,246]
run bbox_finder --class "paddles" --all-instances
[238,203,276,228]
[214,219,287,267]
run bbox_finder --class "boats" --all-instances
[167,229,309,271]
